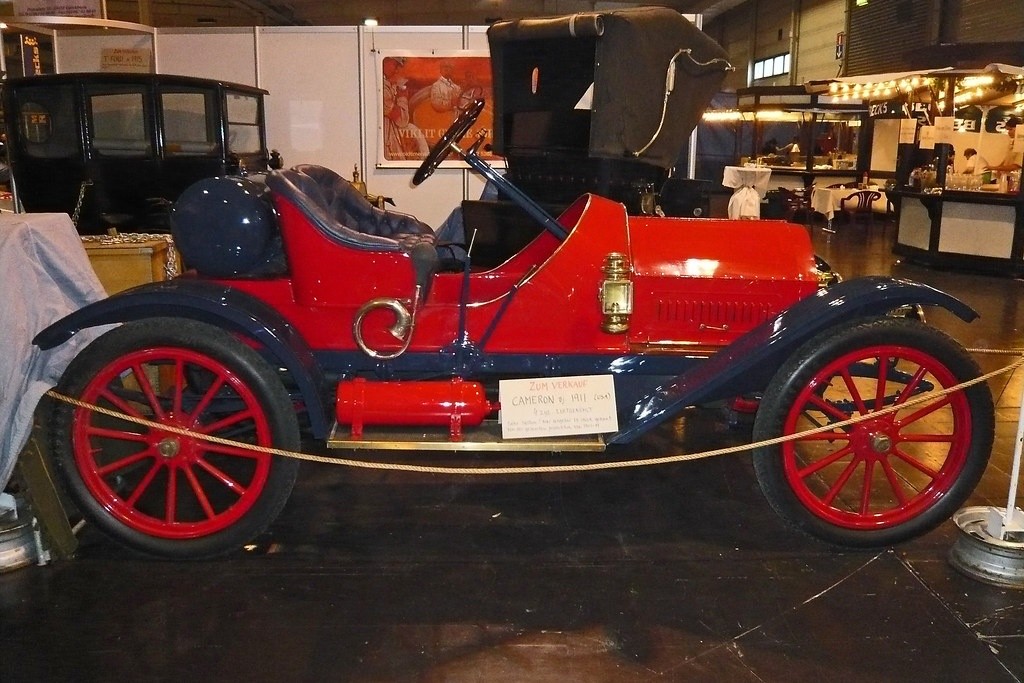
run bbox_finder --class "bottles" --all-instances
[863,172,868,185]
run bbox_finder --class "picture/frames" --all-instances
[375,49,511,168]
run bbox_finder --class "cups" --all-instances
[998,174,1008,193]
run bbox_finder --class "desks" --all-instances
[810,188,896,234]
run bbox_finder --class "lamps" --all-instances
[958,119,966,133]
[790,135,800,153]
[857,0,868,6]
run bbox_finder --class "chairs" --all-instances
[778,187,812,225]
[265,163,441,314]
[836,191,881,227]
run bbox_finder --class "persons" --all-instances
[761,138,777,155]
[945,149,955,174]
[962,148,991,184]
[983,117,1022,193]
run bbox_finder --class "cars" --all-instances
[32,101,997,562]
[1,74,281,229]
